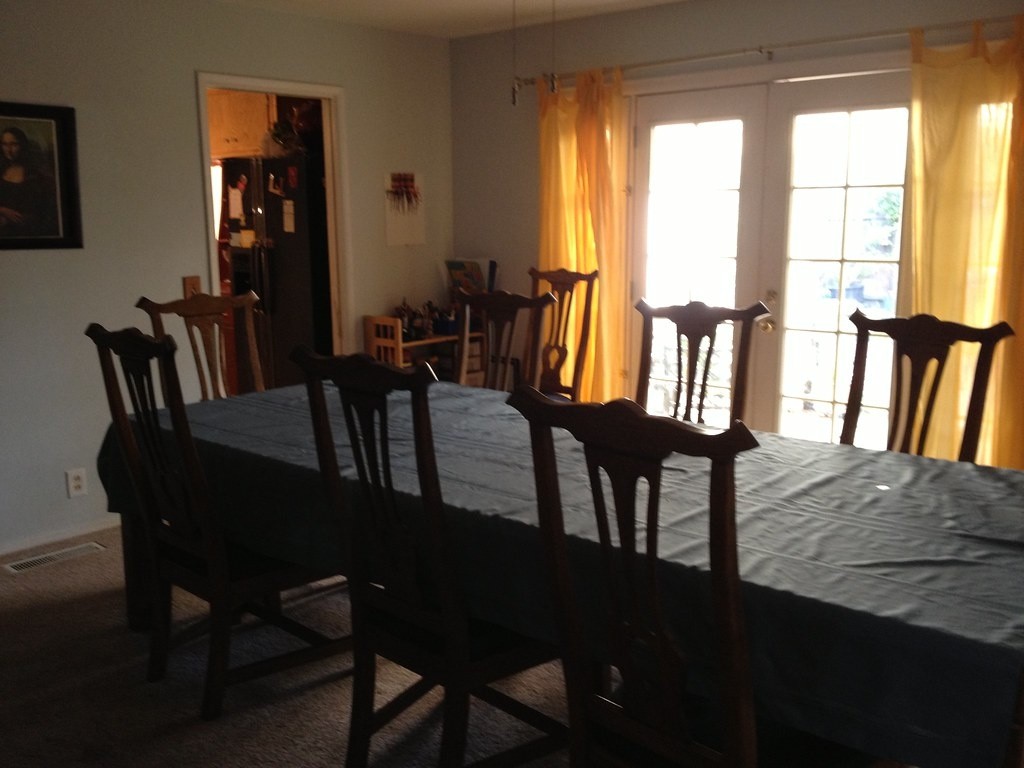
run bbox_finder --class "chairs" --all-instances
[305,354,571,768]
[635,299,769,430]
[840,308,1016,462]
[481,289,557,392]
[528,267,597,401]
[136,294,268,406]
[505,383,761,767]
[85,324,352,717]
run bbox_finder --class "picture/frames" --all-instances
[0,102,82,249]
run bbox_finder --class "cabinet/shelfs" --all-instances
[221,156,324,393]
[362,314,483,385]
[204,89,292,156]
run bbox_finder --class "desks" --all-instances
[96,381,1024,768]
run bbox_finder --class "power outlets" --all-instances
[66,470,86,498]
[183,277,199,299]
